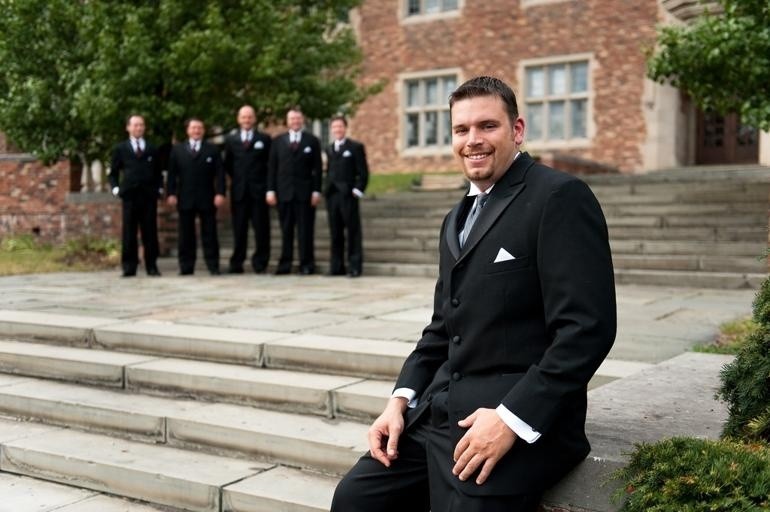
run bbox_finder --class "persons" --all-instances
[324,115,370,277]
[265,105,325,276]
[162,115,227,278]
[331,77,619,511]
[220,105,276,275]
[104,112,171,279]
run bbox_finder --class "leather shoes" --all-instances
[121,261,363,277]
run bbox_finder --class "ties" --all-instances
[135,138,143,157]
[462,194,487,249]
[336,142,341,152]
[191,139,198,155]
[242,132,250,146]
[292,134,299,151]
